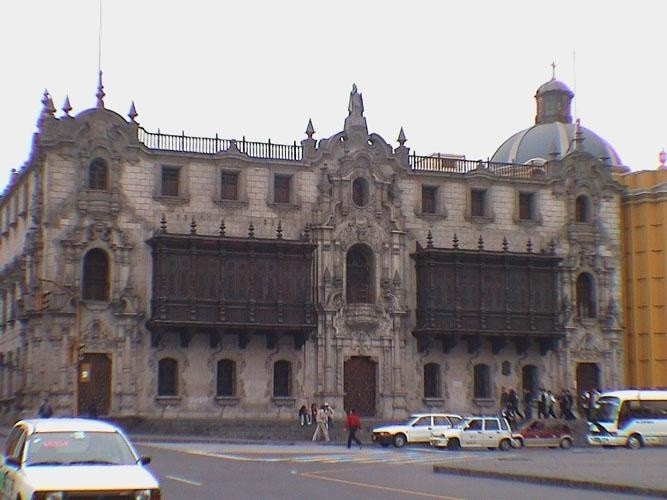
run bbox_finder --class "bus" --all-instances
[586,389,667,450]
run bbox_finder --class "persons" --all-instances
[298,405,311,426]
[312,405,330,441]
[36,398,52,418]
[308,404,318,423]
[499,383,599,420]
[343,409,363,450]
[323,403,334,420]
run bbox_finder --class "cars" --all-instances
[0,417,161,500]
[371,413,576,451]
